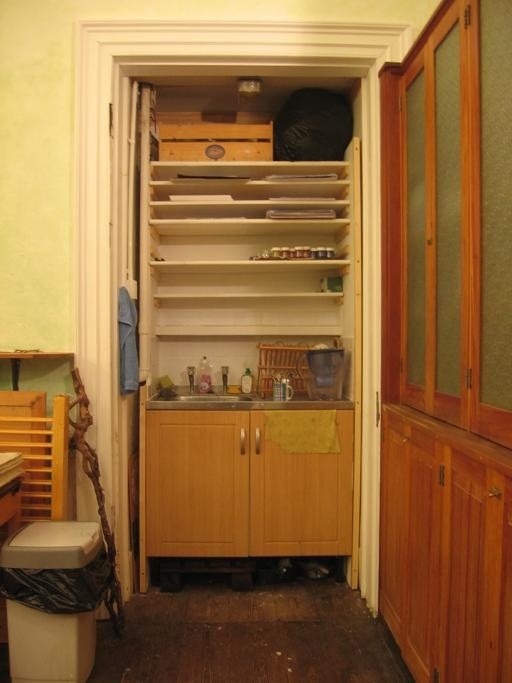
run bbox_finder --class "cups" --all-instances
[272,383,294,401]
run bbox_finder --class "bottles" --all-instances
[271,246,334,259]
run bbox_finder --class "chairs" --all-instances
[1,395,67,529]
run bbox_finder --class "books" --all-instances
[265,173,338,180]
[265,208,336,219]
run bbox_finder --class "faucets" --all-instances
[187,365,196,393]
[220,365,229,393]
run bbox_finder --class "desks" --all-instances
[1,450,24,537]
[0,351,75,393]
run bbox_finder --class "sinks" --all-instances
[169,393,254,411]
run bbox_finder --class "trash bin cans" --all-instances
[1,521,111,682]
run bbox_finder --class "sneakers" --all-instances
[302,562,329,580]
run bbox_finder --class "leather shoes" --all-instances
[279,560,296,580]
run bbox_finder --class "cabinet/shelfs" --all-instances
[138,80,361,594]
[380,407,511,682]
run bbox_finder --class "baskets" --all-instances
[256,336,342,399]
